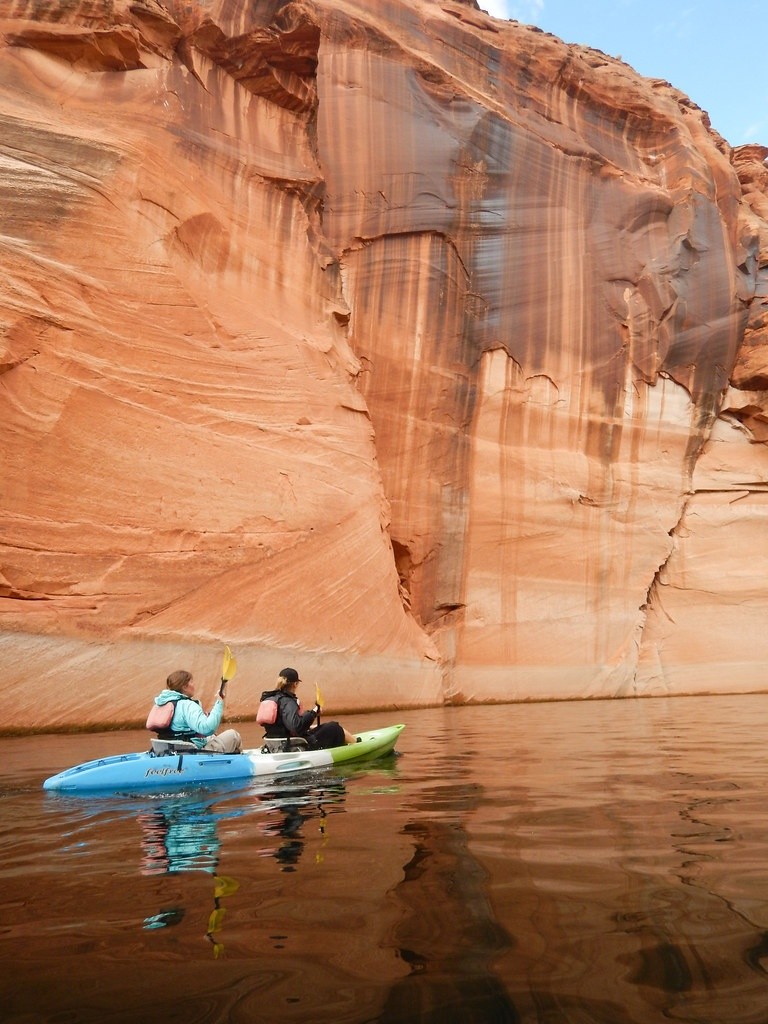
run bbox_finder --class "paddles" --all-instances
[313,680,324,728]
[210,644,237,706]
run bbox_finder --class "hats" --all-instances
[279,668,302,682]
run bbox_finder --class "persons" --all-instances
[145,671,243,756]
[255,667,375,751]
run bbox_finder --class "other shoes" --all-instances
[357,737,374,743]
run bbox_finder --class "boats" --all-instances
[42,722,405,793]
